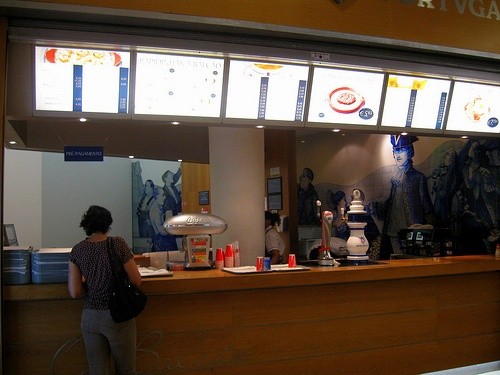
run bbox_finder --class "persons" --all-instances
[265,211,286,265]
[68,205,142,375]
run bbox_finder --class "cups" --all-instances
[288,254,296,268]
[215,240,240,269]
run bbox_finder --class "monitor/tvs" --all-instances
[3,224,20,246]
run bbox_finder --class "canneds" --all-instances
[256,256,271,271]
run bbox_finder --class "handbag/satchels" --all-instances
[107,236,146,323]
